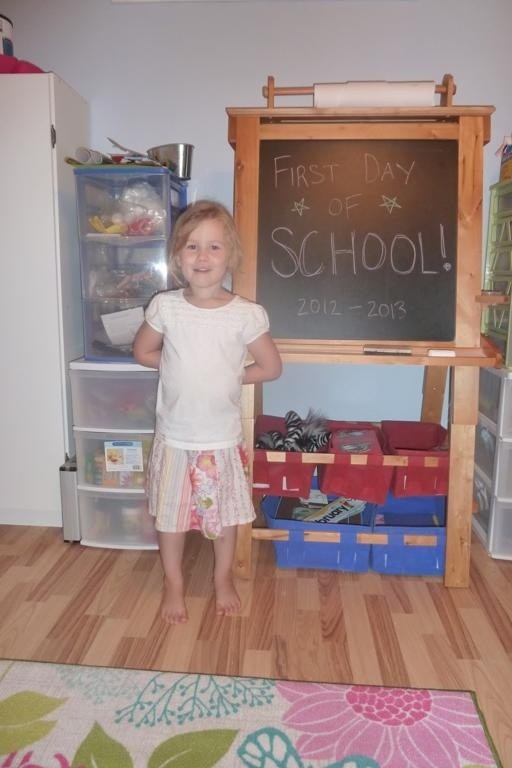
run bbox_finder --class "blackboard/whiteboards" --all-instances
[235,117,482,357]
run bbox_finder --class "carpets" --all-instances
[0,657,503,768]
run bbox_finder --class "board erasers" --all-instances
[363,346,412,356]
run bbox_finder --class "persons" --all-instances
[132,200,282,625]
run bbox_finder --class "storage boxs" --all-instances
[74,166,185,366]
[470,366,510,560]
[255,411,450,578]
[76,489,162,550]
[69,355,158,429]
[73,426,156,491]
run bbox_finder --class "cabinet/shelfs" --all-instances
[0,71,85,530]
